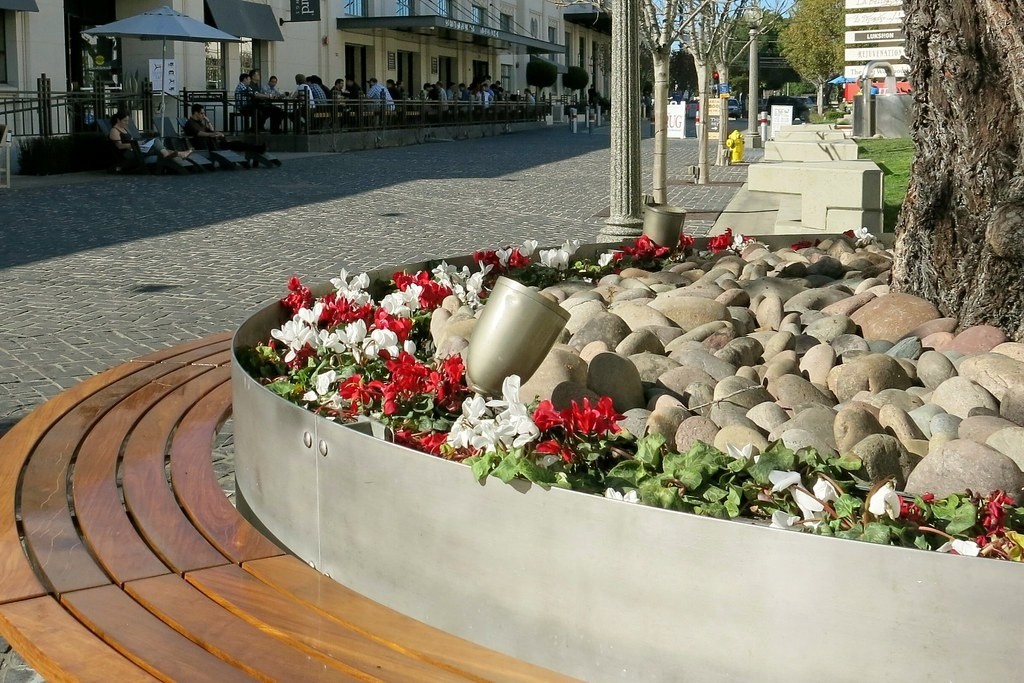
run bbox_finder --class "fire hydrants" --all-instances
[727,130,745,161]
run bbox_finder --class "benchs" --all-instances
[230,108,543,130]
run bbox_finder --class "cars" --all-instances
[758,99,765,112]
[808,95,829,110]
[686,100,699,113]
[728,99,741,119]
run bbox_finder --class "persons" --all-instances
[184,104,272,156]
[233,69,286,133]
[524,88,535,104]
[288,73,364,132]
[365,79,396,131]
[108,110,195,162]
[447,75,506,121]
[386,79,449,124]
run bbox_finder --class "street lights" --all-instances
[744,4,763,148]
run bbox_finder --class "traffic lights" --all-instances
[713,72,719,84]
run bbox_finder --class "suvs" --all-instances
[766,95,816,120]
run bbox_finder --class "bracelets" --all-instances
[207,121,210,123]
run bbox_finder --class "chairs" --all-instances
[95,117,282,176]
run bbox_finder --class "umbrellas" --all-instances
[79,5,245,147]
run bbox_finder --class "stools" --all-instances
[1,328,593,683]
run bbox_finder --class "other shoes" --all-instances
[182,148,193,159]
[163,149,178,160]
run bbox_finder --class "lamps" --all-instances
[465,275,572,397]
[644,202,686,253]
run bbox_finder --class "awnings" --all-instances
[204,0,284,42]
[530,55,568,74]
[0,0,39,12]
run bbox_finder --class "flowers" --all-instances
[237,227,1024,562]
